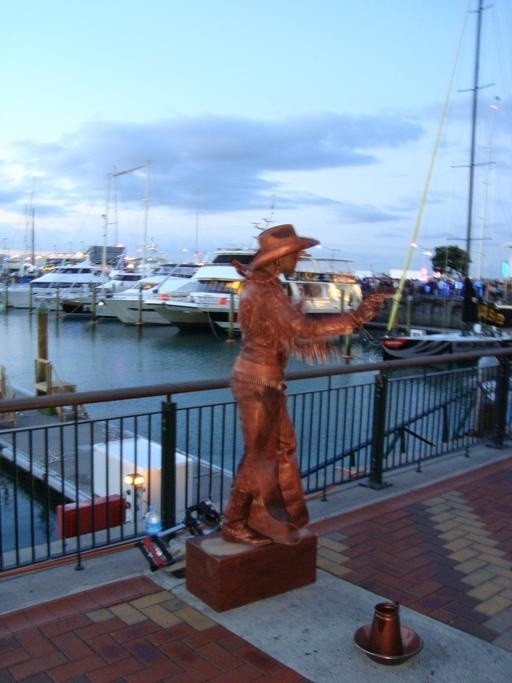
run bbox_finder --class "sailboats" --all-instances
[379,0,512,358]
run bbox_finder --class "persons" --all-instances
[220,221,397,549]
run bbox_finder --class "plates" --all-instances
[358,623,419,659]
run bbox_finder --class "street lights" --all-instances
[411,241,436,256]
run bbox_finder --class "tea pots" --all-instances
[371,602,400,653]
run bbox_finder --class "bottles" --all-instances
[143,504,163,550]
